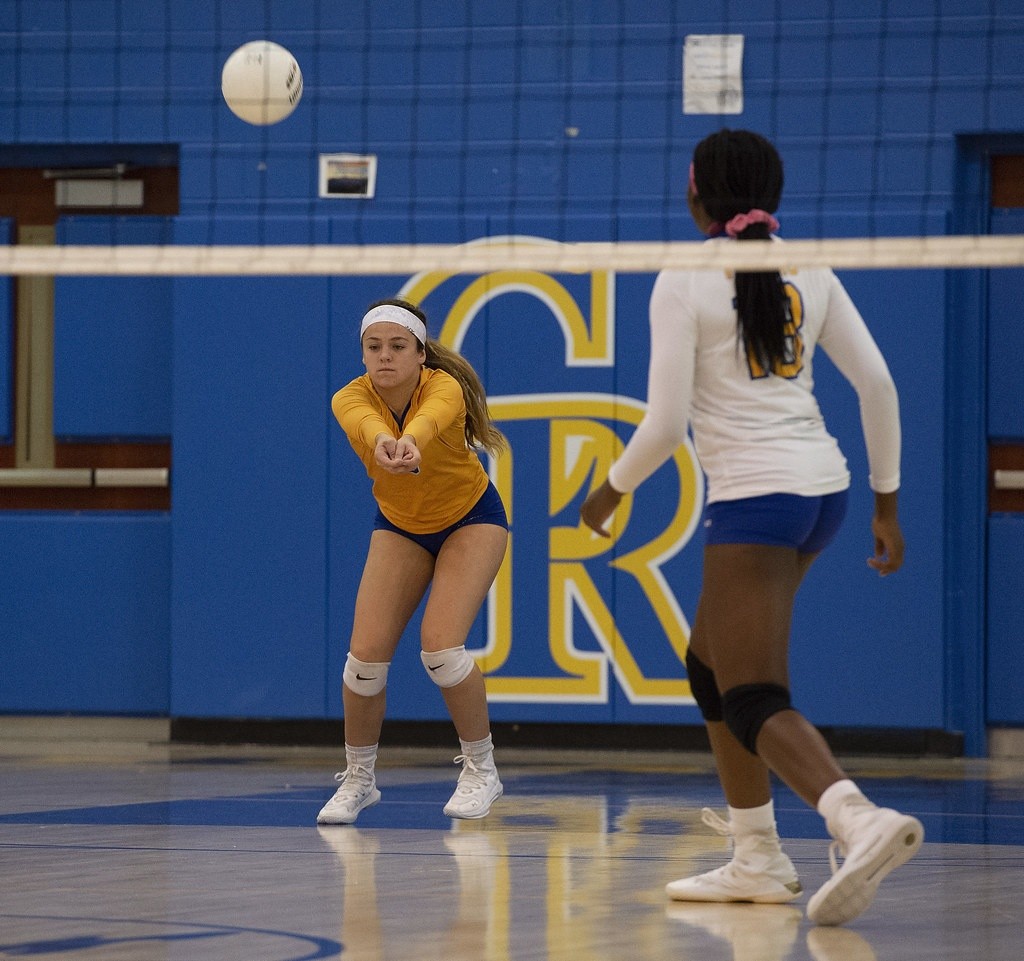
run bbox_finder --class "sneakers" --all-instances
[808,793,925,926]
[318,825,381,856]
[807,927,876,960]
[316,764,381,824]
[665,807,803,901]
[666,902,802,951]
[443,831,495,871]
[442,750,502,820]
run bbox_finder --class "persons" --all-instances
[580,128,924,929]
[316,298,509,824]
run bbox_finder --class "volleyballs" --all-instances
[221,39,304,128]
